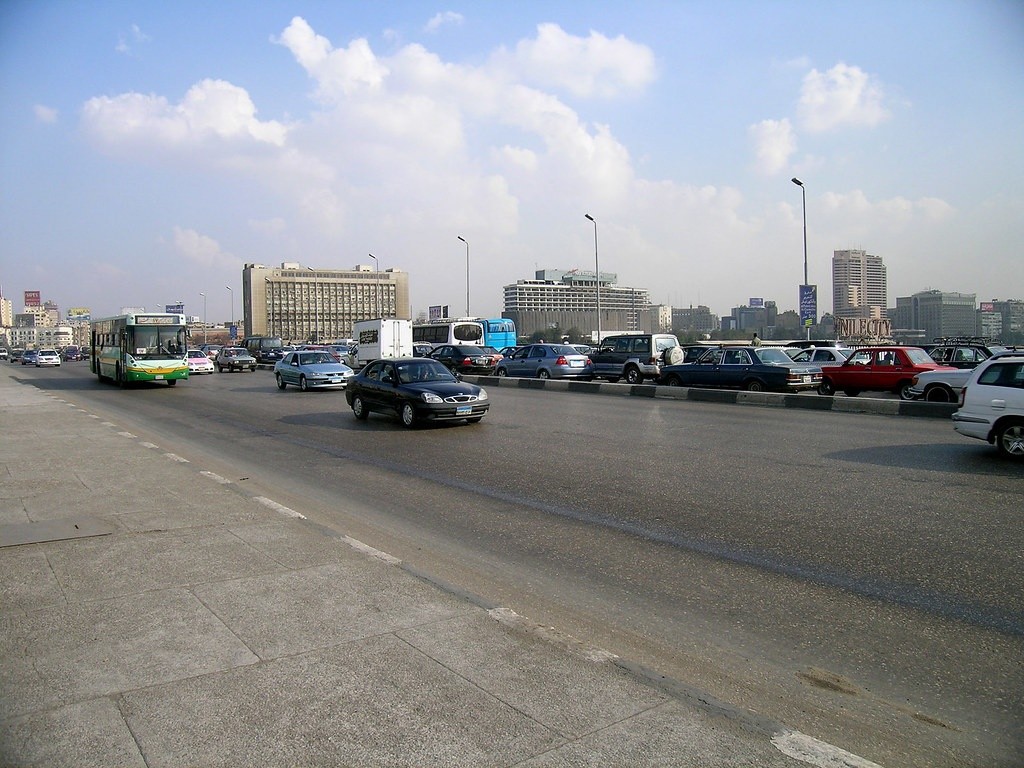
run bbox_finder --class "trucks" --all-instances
[347,318,413,369]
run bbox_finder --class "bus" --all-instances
[89,313,191,387]
[412,317,517,354]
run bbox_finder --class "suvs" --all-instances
[587,334,684,384]
[952,346,1024,455]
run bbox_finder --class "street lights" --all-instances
[585,214,601,345]
[226,286,234,345]
[791,177,810,339]
[308,267,319,344]
[458,236,469,317]
[265,278,273,336]
[200,293,206,343]
[369,253,381,318]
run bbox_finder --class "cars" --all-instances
[818,347,957,401]
[908,336,1024,403]
[187,349,215,375]
[21,350,38,365]
[216,348,258,373]
[413,341,593,381]
[187,336,351,365]
[36,349,61,367]
[0,345,90,363]
[273,350,354,391]
[682,339,871,368]
[660,345,823,394]
[345,357,490,428]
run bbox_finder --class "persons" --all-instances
[752,332,762,347]
[419,368,431,381]
[857,353,879,366]
[956,350,967,362]
[319,355,328,362]
